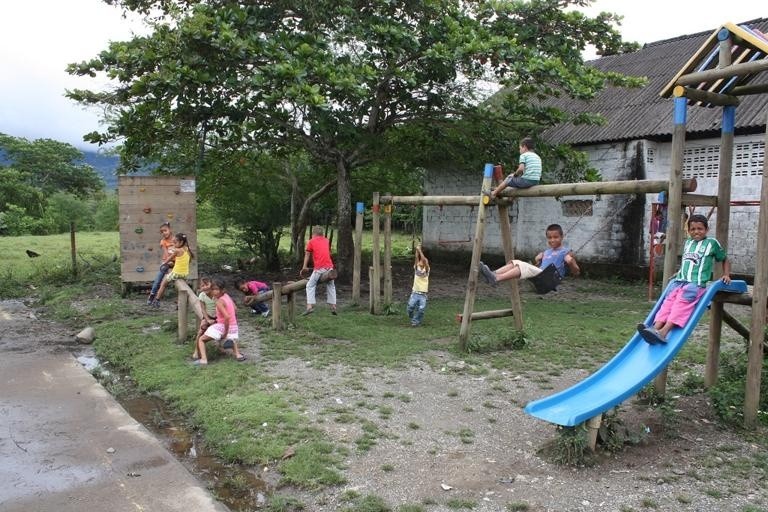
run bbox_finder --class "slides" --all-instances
[524,279,746,426]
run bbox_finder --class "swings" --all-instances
[527,195,636,295]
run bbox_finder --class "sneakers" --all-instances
[480,261,495,287]
[302,308,314,315]
[148,293,161,309]
[251,308,270,318]
[331,307,337,315]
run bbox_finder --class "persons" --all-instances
[404,240,431,328]
[146,222,175,306]
[478,223,580,294]
[152,232,195,309]
[480,137,542,201]
[681,213,690,236]
[298,225,338,317]
[635,214,731,347]
[647,209,663,258]
[193,276,249,367]
[233,277,271,318]
[190,275,217,362]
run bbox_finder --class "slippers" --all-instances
[482,188,495,200]
[638,323,667,345]
[192,348,247,366]
